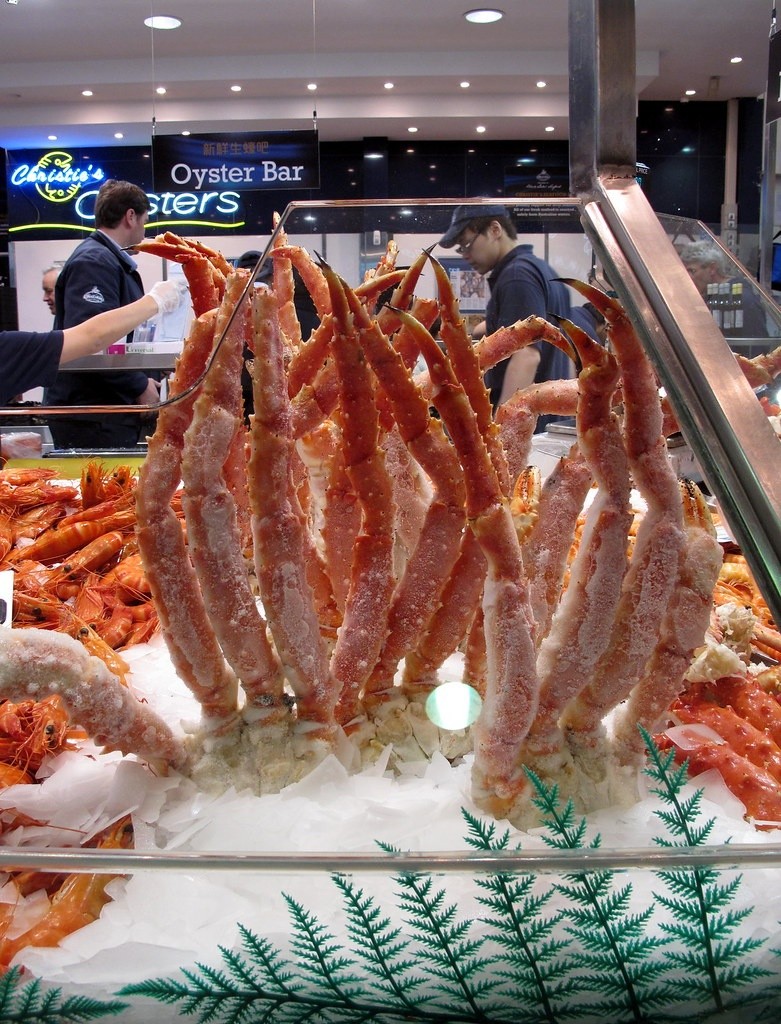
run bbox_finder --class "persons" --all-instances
[41,265,66,317]
[43,180,162,449]
[438,196,619,436]
[681,241,775,405]
[0,279,190,408]
[230,250,441,433]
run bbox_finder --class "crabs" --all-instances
[2,225,781,833]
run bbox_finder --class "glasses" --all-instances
[456,227,484,254]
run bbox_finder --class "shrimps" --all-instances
[0,469,187,973]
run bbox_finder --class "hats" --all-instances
[438,197,507,250]
[234,250,271,272]
